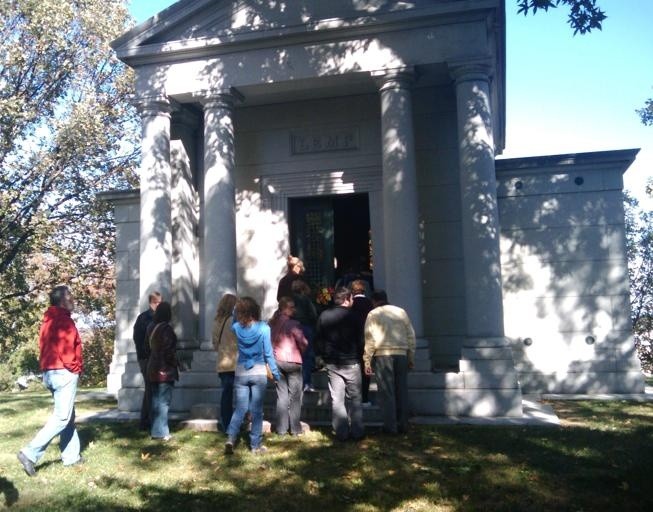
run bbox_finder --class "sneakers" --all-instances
[225,435,234,454]
[303,383,315,392]
[17,452,36,477]
[362,401,372,407]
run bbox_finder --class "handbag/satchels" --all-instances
[266,362,273,380]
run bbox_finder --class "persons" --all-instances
[265,296,311,440]
[314,284,368,439]
[15,285,84,477]
[142,300,179,439]
[220,295,281,455]
[363,291,418,441]
[273,254,369,399]
[130,292,166,427]
[207,293,240,432]
